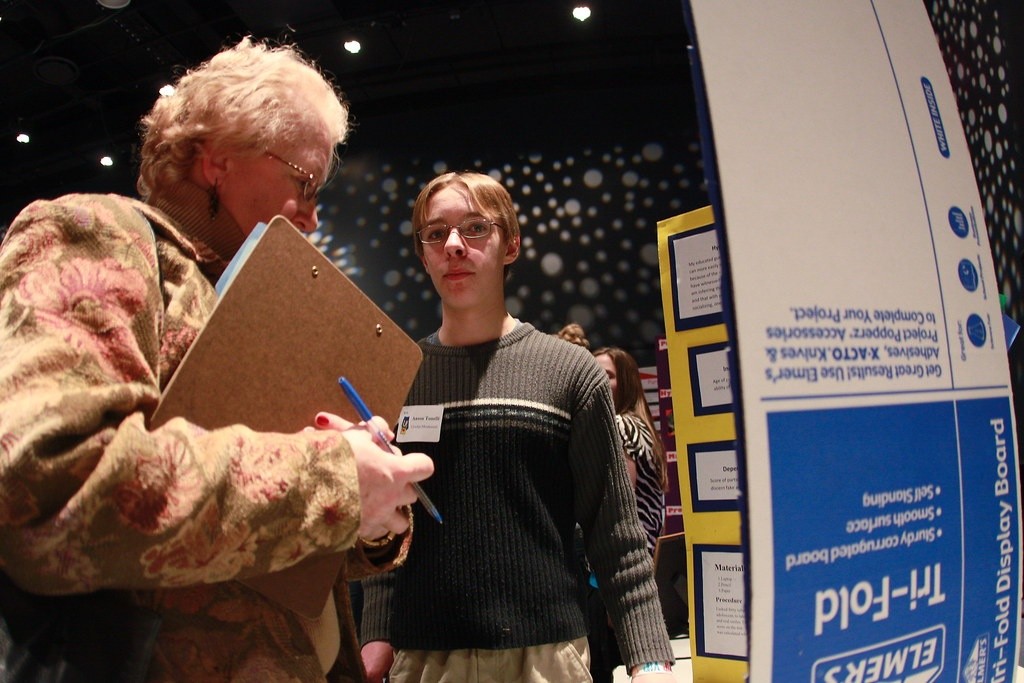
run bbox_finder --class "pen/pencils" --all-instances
[336,376,442,524]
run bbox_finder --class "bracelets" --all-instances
[359,530,395,547]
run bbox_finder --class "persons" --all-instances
[355,174,679,683]
[1,37,433,683]
[551,319,666,683]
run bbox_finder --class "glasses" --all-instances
[417,217,507,243]
[265,151,324,203]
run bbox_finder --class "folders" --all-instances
[146,213,426,623]
